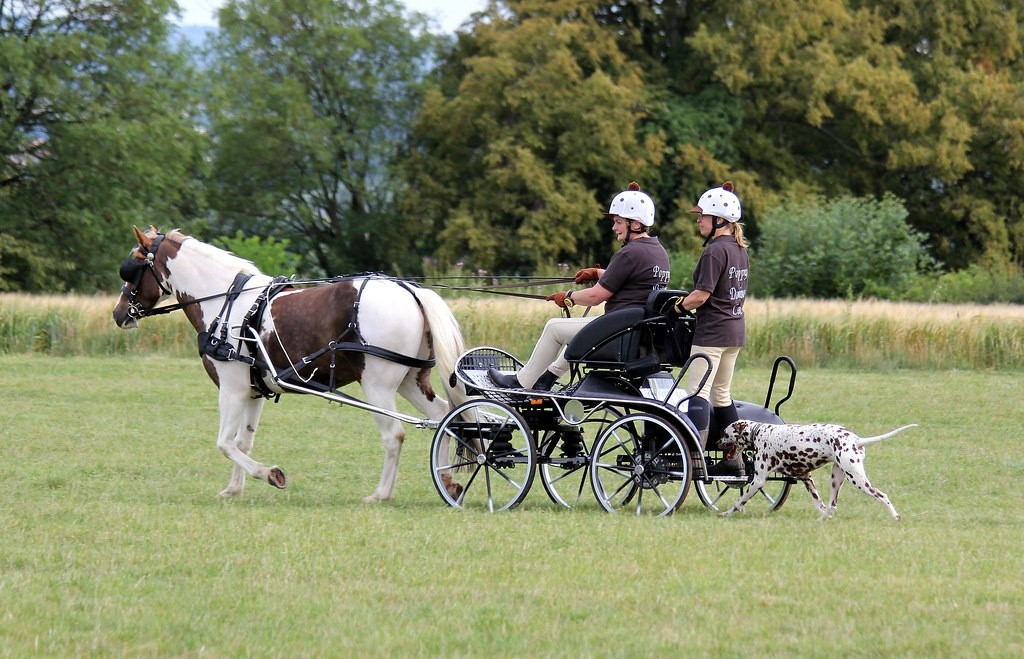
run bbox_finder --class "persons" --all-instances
[486,182,671,403]
[669,180,750,479]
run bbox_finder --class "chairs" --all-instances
[563,289,697,385]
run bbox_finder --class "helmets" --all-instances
[602,182,656,227]
[689,181,742,223]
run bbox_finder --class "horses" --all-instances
[112,222,490,505]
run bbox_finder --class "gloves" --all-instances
[575,267,600,285]
[546,292,566,307]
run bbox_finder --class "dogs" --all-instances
[716,418,919,523]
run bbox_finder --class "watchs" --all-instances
[674,296,689,315]
[561,290,576,309]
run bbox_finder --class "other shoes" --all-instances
[707,452,747,476]
[532,369,559,392]
[670,452,706,480]
[487,368,528,401]
